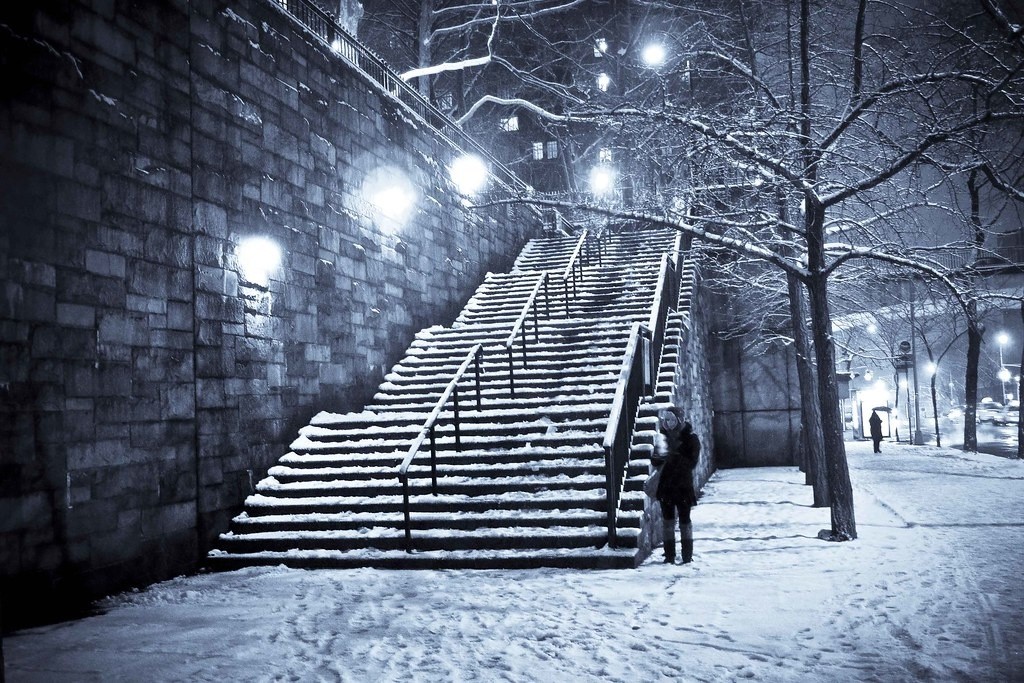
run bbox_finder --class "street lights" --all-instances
[997,332,1010,404]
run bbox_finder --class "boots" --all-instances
[663,539,675,564]
[682,538,694,563]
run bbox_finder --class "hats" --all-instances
[658,407,685,433]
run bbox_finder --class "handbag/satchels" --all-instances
[642,460,668,500]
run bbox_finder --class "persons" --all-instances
[650,405,701,563]
[868,410,882,454]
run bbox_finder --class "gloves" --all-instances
[651,456,664,467]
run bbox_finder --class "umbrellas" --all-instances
[871,406,892,412]
